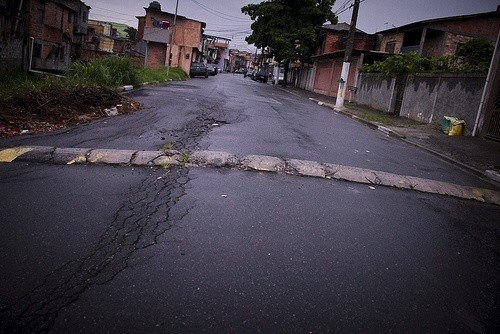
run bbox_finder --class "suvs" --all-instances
[189,63,208,78]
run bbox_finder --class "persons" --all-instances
[61,25,70,56]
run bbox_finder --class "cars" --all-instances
[206,64,221,76]
[247,71,268,83]
[272,72,284,83]
